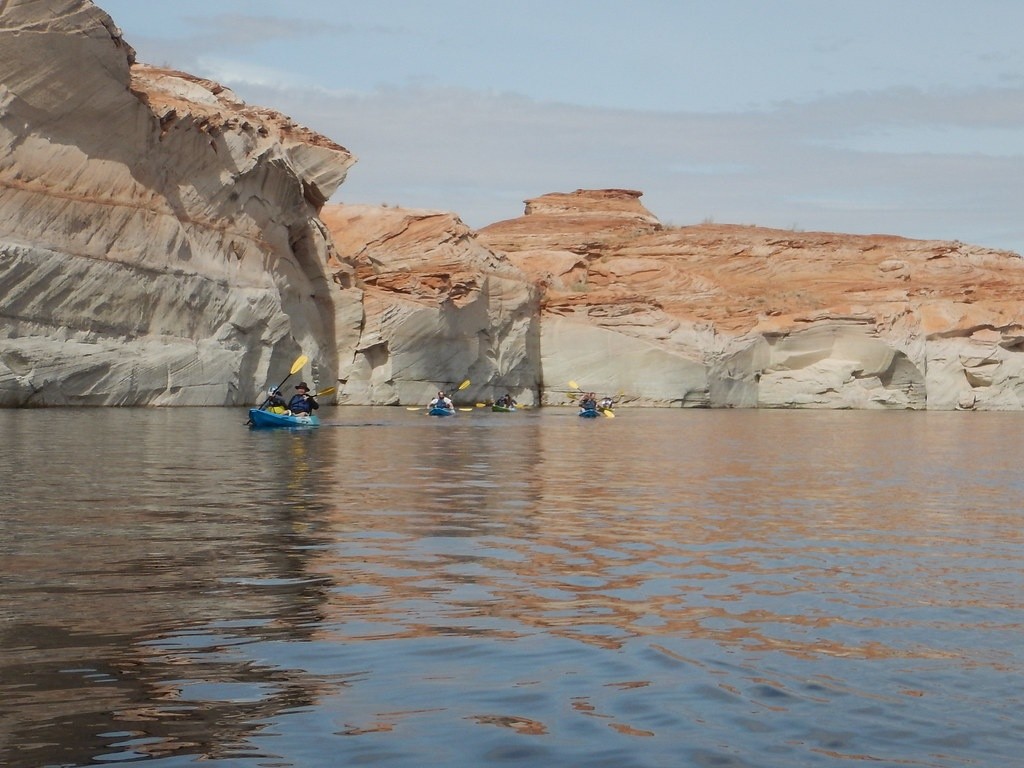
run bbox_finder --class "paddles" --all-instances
[246,354,308,424]
[568,379,616,418]
[476,403,524,408]
[568,393,614,414]
[448,380,471,398]
[285,387,335,409]
[406,407,473,412]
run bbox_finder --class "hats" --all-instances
[294,382,310,392]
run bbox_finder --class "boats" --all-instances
[429,406,455,417]
[490,404,518,412]
[577,408,600,418]
[248,408,321,429]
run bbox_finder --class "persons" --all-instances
[502,393,518,408]
[282,382,320,418]
[578,392,597,410]
[426,391,454,410]
[266,385,289,416]
[495,396,505,407]
